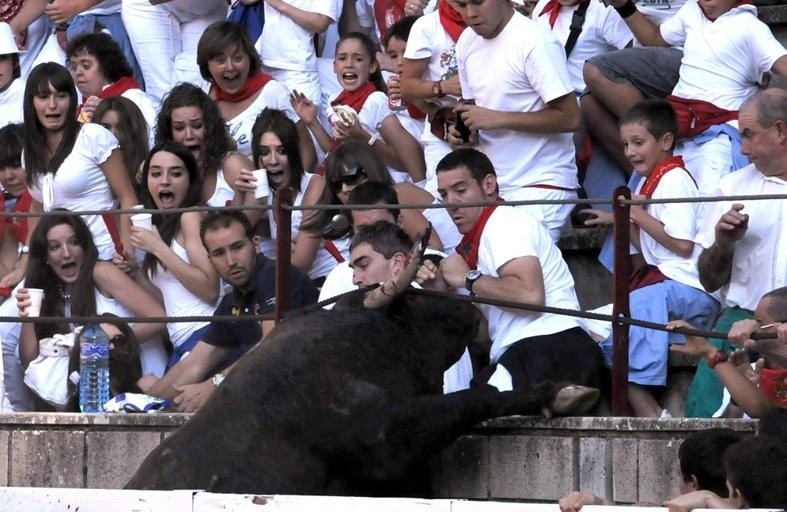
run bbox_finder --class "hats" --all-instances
[0,22,30,55]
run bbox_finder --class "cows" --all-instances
[122,221,601,498]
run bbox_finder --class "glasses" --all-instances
[328,164,364,194]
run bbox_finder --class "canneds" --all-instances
[387,76,407,111]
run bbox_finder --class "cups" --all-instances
[247,169,270,199]
[20,288,44,318]
[130,214,152,231]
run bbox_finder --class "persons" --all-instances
[759,408,787,437]
[0,0,787,418]
[664,436,787,512]
[557,428,743,512]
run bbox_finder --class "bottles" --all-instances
[78,313,111,414]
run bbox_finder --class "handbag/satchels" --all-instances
[67,314,144,412]
